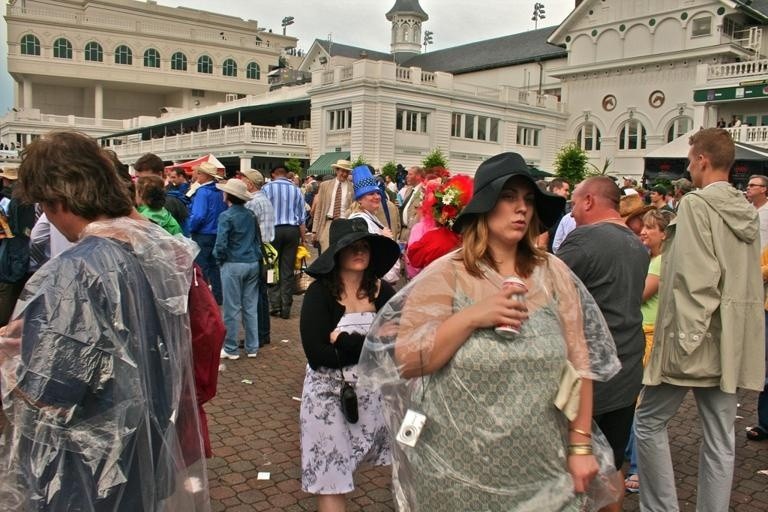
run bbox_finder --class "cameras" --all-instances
[395,408,427,448]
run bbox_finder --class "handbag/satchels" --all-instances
[262,243,280,286]
[296,257,316,294]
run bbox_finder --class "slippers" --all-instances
[624,477,639,493]
[747,426,768,440]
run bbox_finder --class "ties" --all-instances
[333,183,341,218]
[399,190,414,226]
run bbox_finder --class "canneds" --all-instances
[495,276,528,339]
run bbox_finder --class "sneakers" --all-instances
[220,348,240,360]
[270,309,289,318]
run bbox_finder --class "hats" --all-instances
[331,159,353,170]
[192,162,264,200]
[352,165,380,200]
[452,152,566,234]
[304,217,400,278]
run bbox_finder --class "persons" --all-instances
[1,129,306,511]
[300,116,767,511]
